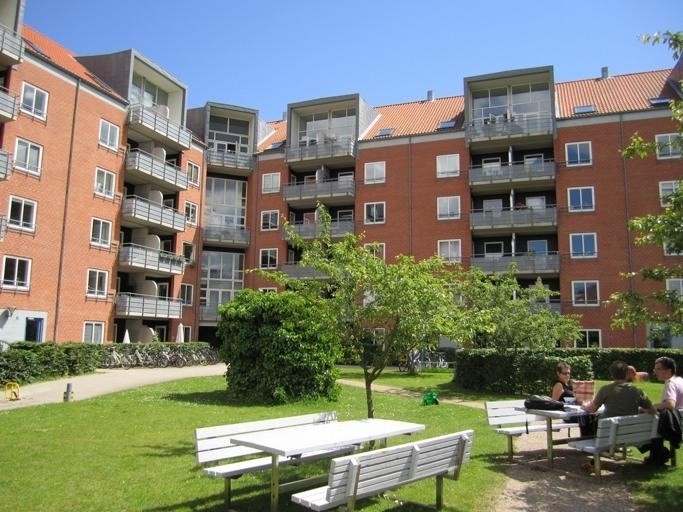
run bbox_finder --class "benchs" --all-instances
[193,408,474,512]
[484,386,683,477]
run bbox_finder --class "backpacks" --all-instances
[524,394,570,412]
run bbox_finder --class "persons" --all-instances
[633,356,683,467]
[551,361,599,440]
[625,365,636,385]
[580,360,658,470]
[408,346,420,370]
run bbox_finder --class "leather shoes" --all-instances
[644,446,671,466]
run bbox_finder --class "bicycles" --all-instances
[397,349,450,372]
[98,343,220,369]
